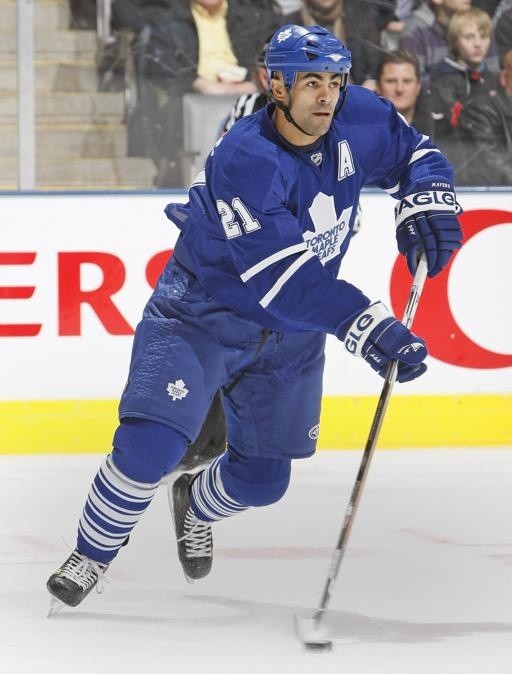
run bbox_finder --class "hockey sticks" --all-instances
[295,249,427,646]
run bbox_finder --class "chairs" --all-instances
[178,90,251,189]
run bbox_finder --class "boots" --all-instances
[47,534,129,606]
[172,469,212,578]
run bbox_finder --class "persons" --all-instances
[69,1,512,189]
[46,21,464,608]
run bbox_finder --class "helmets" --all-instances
[257,23,353,91]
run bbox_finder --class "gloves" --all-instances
[394,176,462,279]
[335,301,427,383]
